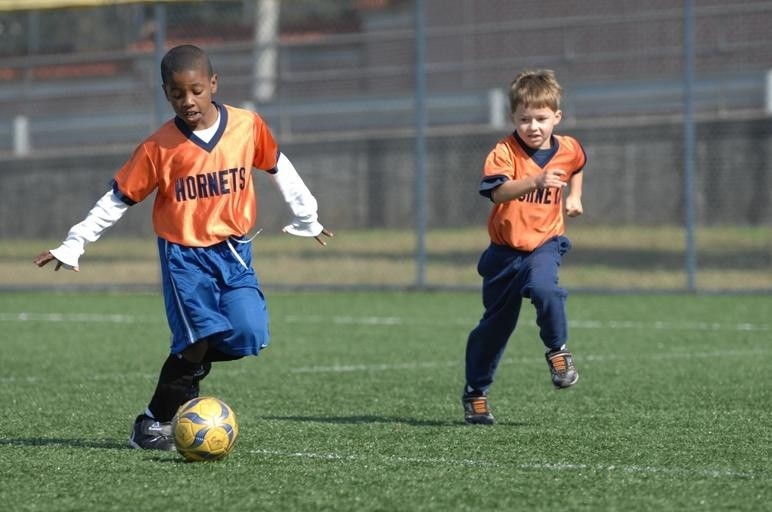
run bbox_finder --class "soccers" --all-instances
[171,396,238,461]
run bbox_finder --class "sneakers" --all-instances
[460,385,496,425]
[180,362,212,404]
[128,412,180,453]
[545,345,580,389]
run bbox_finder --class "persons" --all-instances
[461,67,587,425]
[33,44,335,451]
